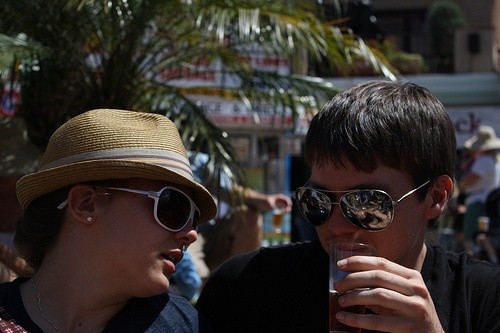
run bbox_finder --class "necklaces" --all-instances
[30,278,60,333]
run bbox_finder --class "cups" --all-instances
[356,209,375,225]
[329,242,376,333]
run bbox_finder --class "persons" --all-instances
[188,151,292,272]
[459,124,500,237]
[0,108,218,333]
[195,80,500,333]
[452,148,474,231]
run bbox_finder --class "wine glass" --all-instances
[272,202,283,234]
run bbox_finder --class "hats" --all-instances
[16,108,218,226]
[463,125,500,153]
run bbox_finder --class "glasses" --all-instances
[57,186,201,232]
[292,179,431,233]
